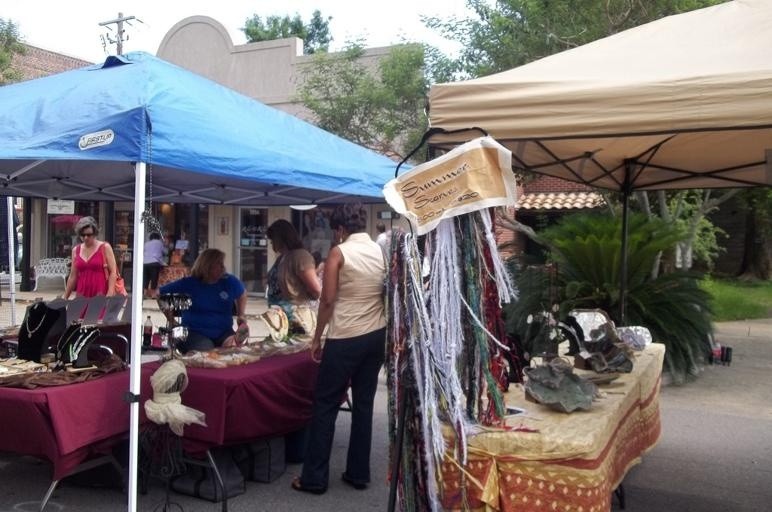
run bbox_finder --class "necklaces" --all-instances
[26,310,48,337]
[66,328,96,363]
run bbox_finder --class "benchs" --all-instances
[33,256,72,292]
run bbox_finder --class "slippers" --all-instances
[291,470,366,493]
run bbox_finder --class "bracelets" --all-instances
[235,318,244,324]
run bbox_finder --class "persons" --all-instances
[58,324,102,369]
[265,219,321,336]
[313,247,326,281]
[19,302,66,363]
[375,219,392,261]
[155,247,247,356]
[143,233,166,299]
[291,201,389,495]
[61,215,115,321]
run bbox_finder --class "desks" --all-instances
[2,360,162,511]
[162,338,353,509]
[431,340,666,512]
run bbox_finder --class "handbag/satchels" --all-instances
[114,278,127,297]
